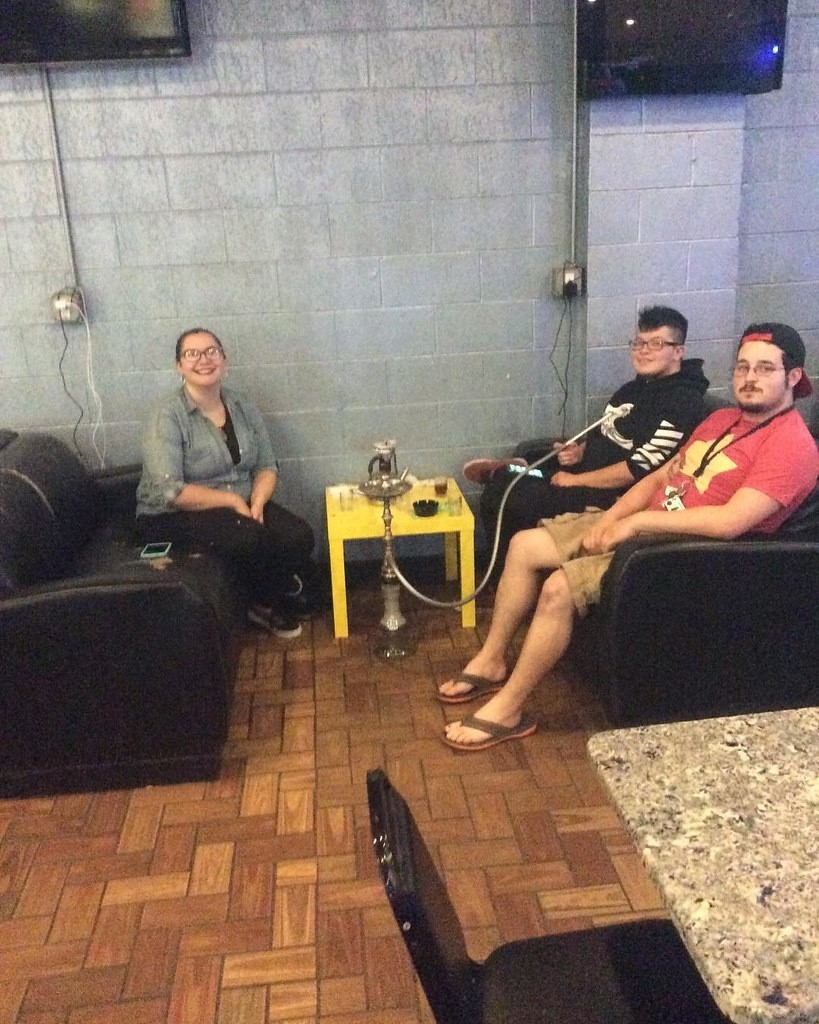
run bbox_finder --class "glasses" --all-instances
[628,336,678,351]
[734,364,784,376]
[179,347,221,361]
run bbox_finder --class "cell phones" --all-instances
[141,542,171,558]
[507,464,545,479]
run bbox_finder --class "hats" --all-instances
[738,324,812,398]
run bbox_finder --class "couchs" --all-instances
[509,394,819,728]
[0,428,249,799]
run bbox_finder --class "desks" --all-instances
[325,479,475,637]
[586,706,819,1024]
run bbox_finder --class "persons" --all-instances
[133,329,316,639]
[453,304,711,615]
[433,322,819,752]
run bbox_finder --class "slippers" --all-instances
[439,711,537,750]
[434,673,509,702]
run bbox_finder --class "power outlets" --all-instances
[553,268,584,298]
[53,294,84,324]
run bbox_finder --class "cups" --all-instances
[448,496,462,516]
[340,487,354,511]
[434,476,448,497]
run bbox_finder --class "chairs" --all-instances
[366,767,735,1024]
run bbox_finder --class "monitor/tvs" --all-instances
[576,0,788,99]
[0,0,192,63]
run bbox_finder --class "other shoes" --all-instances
[461,456,528,483]
[246,600,301,638]
[453,589,493,613]
[284,598,312,620]
